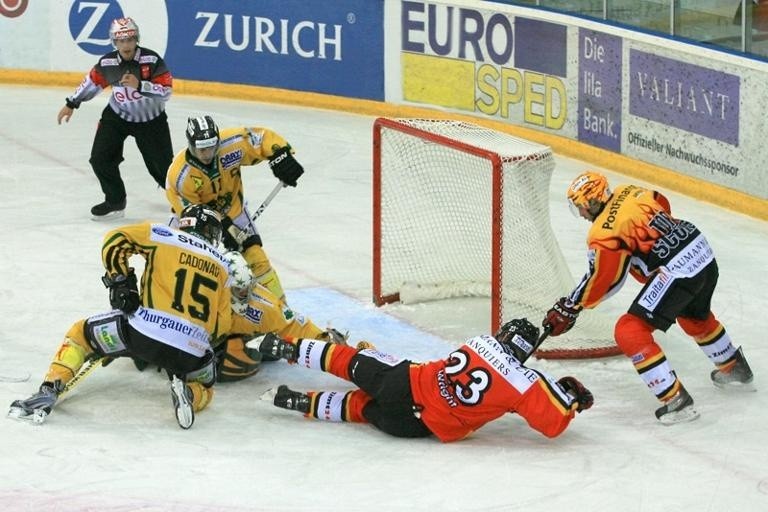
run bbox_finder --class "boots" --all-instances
[10,379,65,415]
[171,379,193,428]
[259,332,296,360]
[655,371,694,420]
[274,385,310,414]
[710,347,754,383]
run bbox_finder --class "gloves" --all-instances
[102,268,139,312]
[542,298,582,335]
[269,146,303,187]
[559,377,594,409]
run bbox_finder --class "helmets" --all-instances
[186,116,219,157]
[567,171,611,220]
[222,250,255,316]
[110,18,140,49]
[496,318,539,363]
[180,203,223,248]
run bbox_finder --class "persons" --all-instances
[259,318,594,444]
[165,114,304,309]
[216,251,348,383]
[11,203,232,428]
[542,172,753,420]
[57,16,176,216]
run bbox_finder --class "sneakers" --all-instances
[91,200,127,215]
